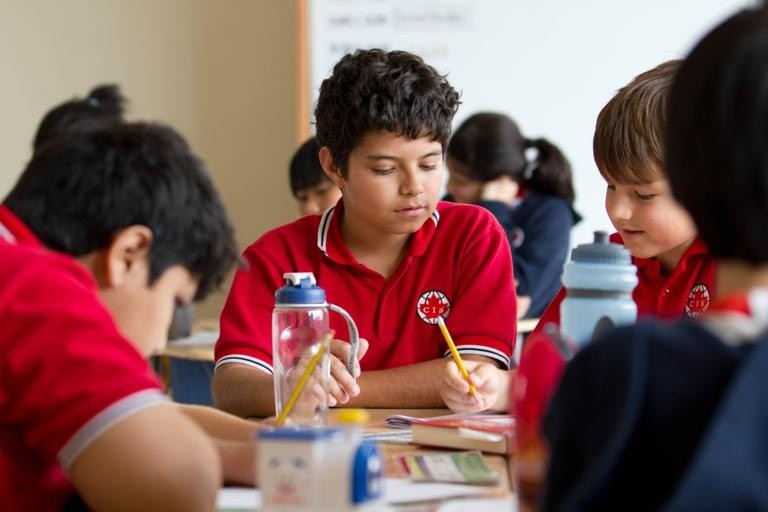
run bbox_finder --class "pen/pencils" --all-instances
[437,314,480,403]
[271,331,337,434]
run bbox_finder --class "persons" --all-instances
[0,120,272,511]
[31,85,193,343]
[439,112,582,320]
[211,48,518,418]
[288,135,344,216]
[540,4,765,511]
[590,60,718,319]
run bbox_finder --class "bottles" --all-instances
[272,271,333,430]
[561,231,636,356]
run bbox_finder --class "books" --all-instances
[411,412,514,457]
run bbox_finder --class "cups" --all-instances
[255,425,383,512]
